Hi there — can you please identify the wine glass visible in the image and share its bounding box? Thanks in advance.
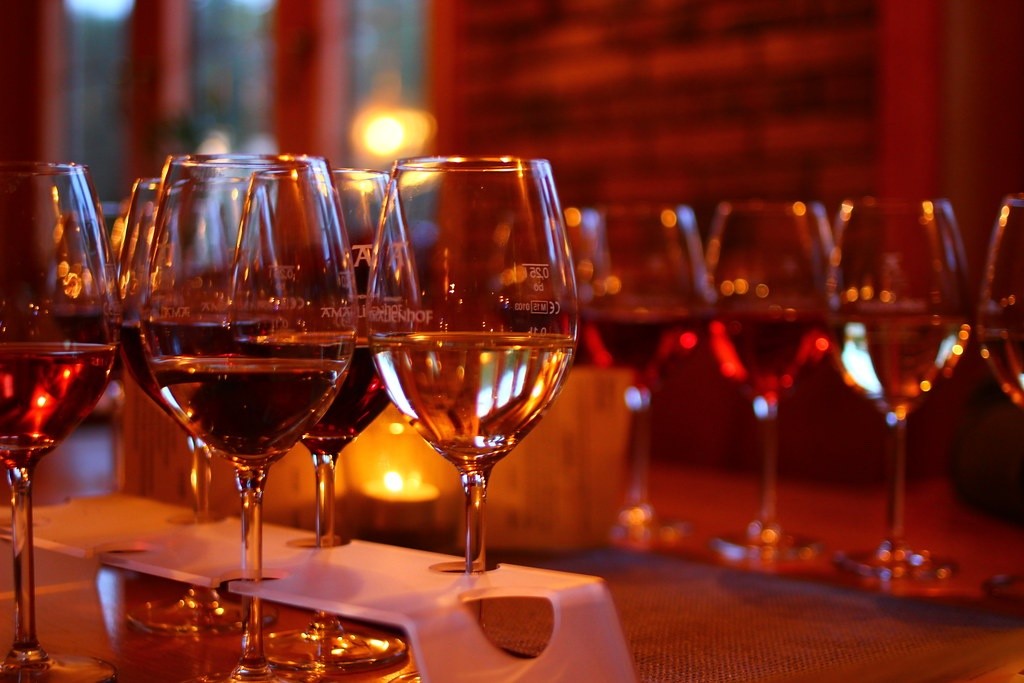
[571,202,708,547]
[366,154,582,575]
[0,160,123,683]
[104,150,421,683]
[976,193,1024,603]
[704,197,840,562]
[826,195,974,582]
